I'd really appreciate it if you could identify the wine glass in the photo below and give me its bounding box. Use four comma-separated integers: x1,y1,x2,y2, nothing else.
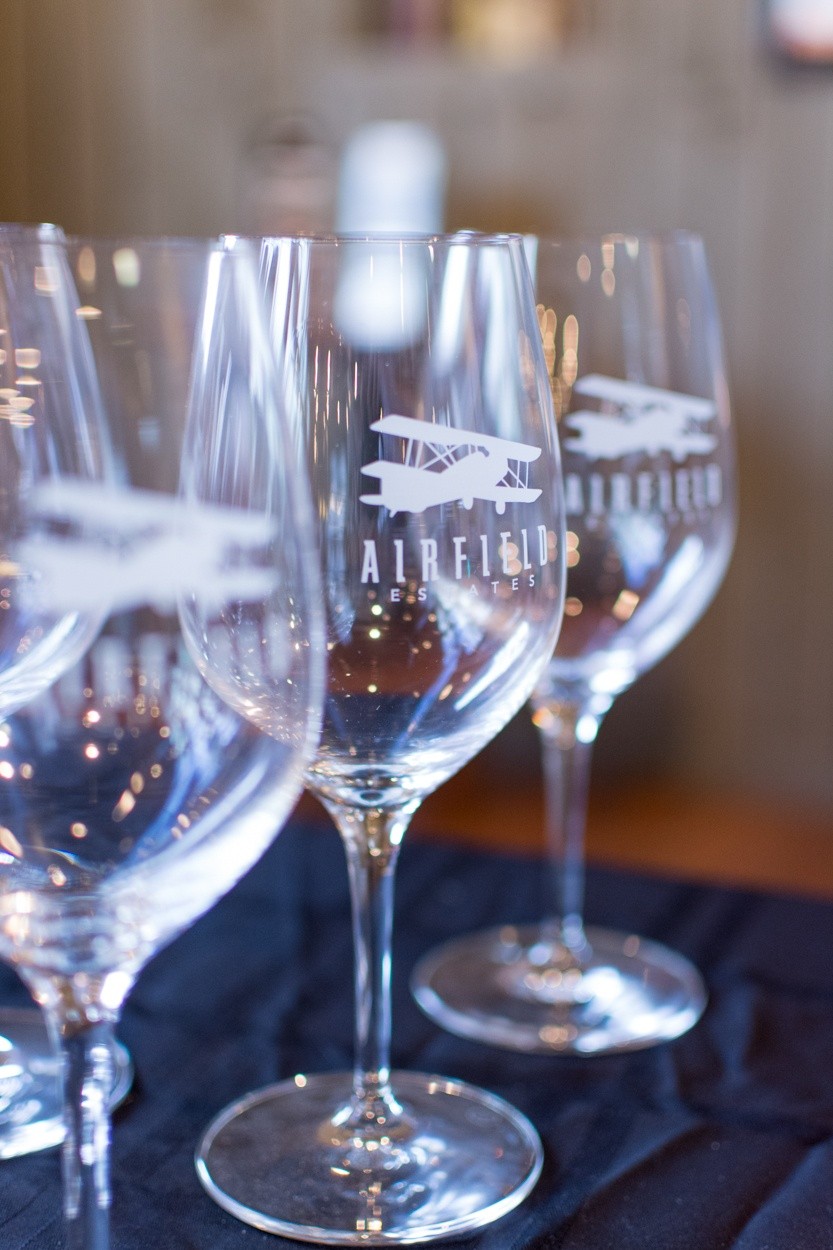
0,215,742,1250
